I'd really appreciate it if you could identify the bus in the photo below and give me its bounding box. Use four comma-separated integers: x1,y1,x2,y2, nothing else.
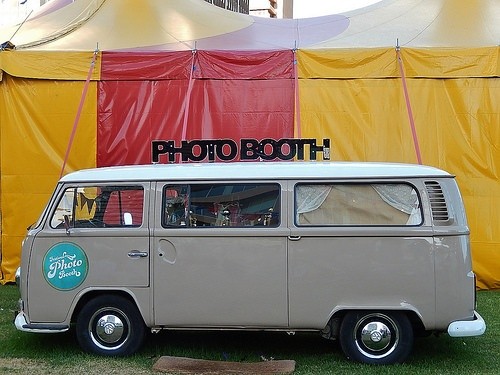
14,163,487,366
